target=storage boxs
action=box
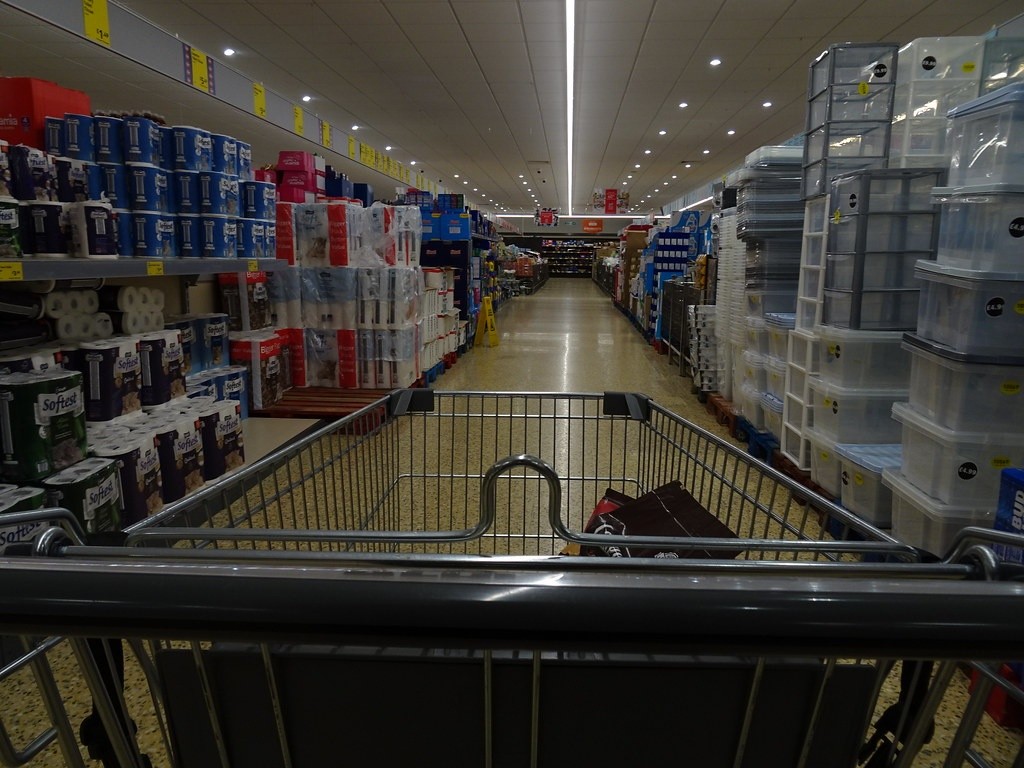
[0,76,92,156]
[254,150,486,321]
[688,36,1024,565]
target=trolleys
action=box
[1,388,1023,768]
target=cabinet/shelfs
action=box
[541,245,595,279]
